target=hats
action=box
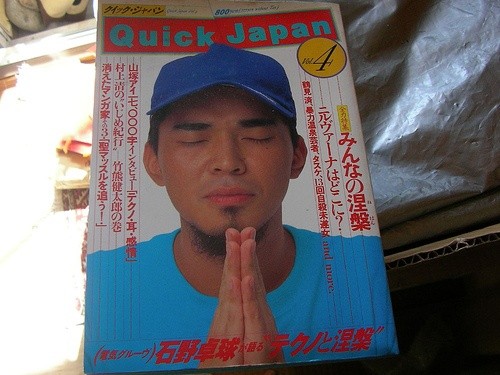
[147,42,298,124]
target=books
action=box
[83,0,400,375]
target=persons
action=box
[83,43,399,375]
